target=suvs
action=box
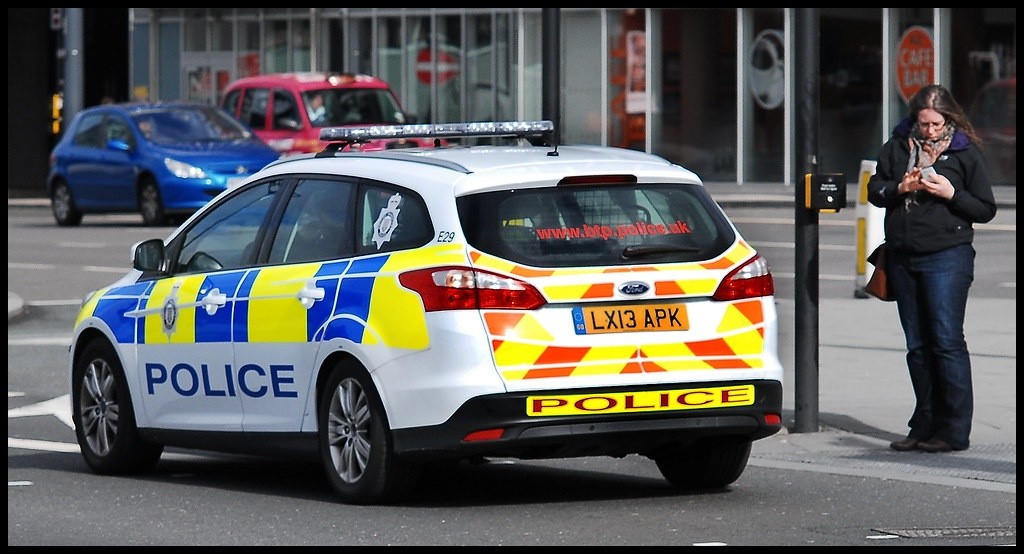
[219,71,452,160]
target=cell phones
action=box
[921,166,938,183]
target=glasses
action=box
[916,118,950,131]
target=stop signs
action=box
[417,49,457,85]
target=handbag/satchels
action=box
[864,242,897,302]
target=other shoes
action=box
[890,437,920,451]
[917,437,957,452]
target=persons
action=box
[868,84,996,453]
[126,117,153,144]
[306,93,326,123]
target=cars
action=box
[46,99,301,229]
[967,77,1015,159]
[67,118,787,506]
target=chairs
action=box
[500,194,546,257]
